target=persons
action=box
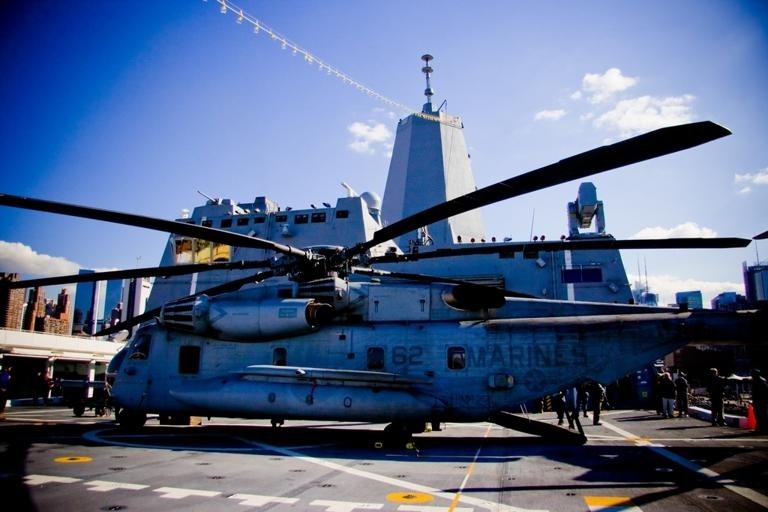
[552,382,609,427]
[653,373,663,416]
[659,372,676,417]
[705,368,727,428]
[674,372,690,418]
[748,370,766,435]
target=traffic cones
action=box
[747,405,758,430]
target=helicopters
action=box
[0,120,752,453]
